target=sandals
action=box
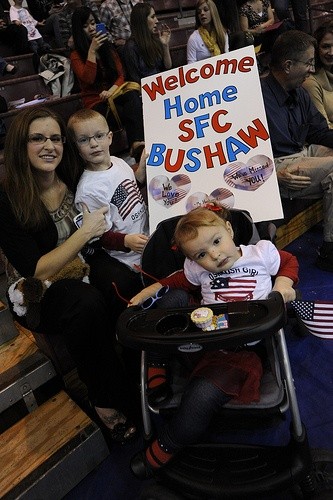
[90,403,138,444]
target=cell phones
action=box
[73,212,99,244]
[96,24,105,37]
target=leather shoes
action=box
[146,362,173,408]
[128,440,187,481]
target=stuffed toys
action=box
[5,255,89,320]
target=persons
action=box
[127,207,300,479]
[0,0,333,163]
[260,30,333,272]
[0,107,144,441]
[66,109,149,278]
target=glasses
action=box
[27,134,66,144]
[290,57,315,69]
[73,130,110,145]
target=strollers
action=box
[117,206,329,497]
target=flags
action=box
[290,299,333,340]
